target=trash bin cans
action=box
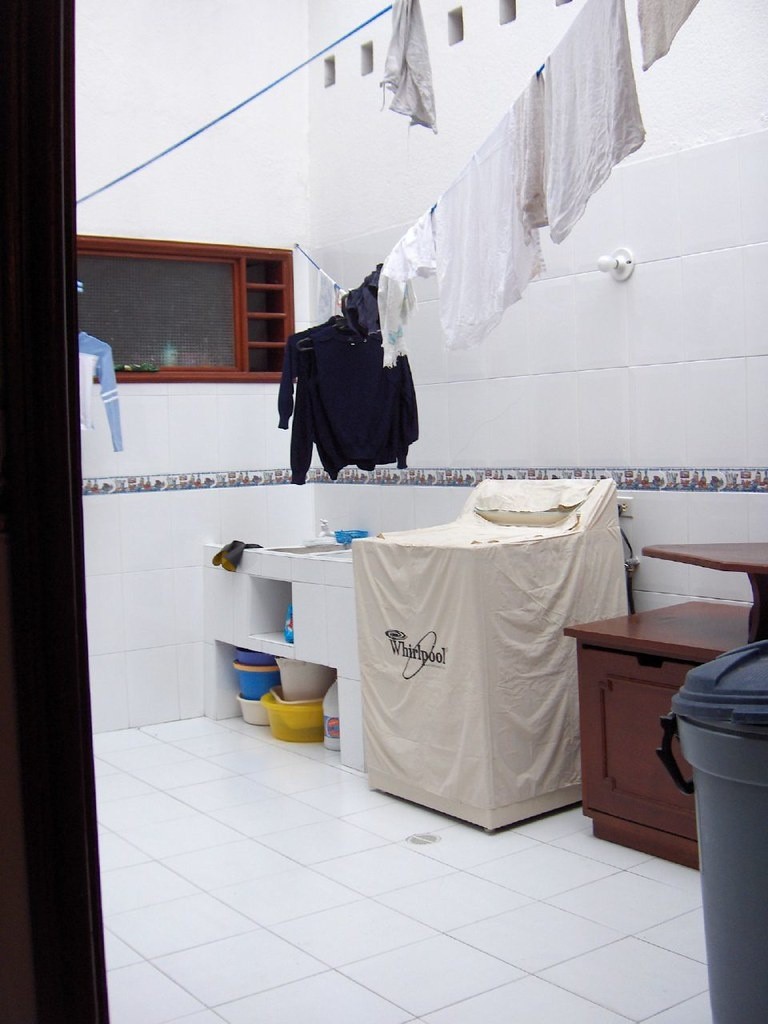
[656,639,768,1024]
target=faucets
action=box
[318,518,336,539]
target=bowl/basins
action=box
[236,687,324,742]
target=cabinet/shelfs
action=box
[563,542,768,869]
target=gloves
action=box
[211,540,264,571]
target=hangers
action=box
[296,288,360,352]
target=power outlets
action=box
[618,496,634,519]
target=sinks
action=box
[264,543,353,554]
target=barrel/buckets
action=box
[656,639,768,1024]
[233,659,282,701]
[322,678,341,750]
[236,647,277,666]
[275,658,337,701]
